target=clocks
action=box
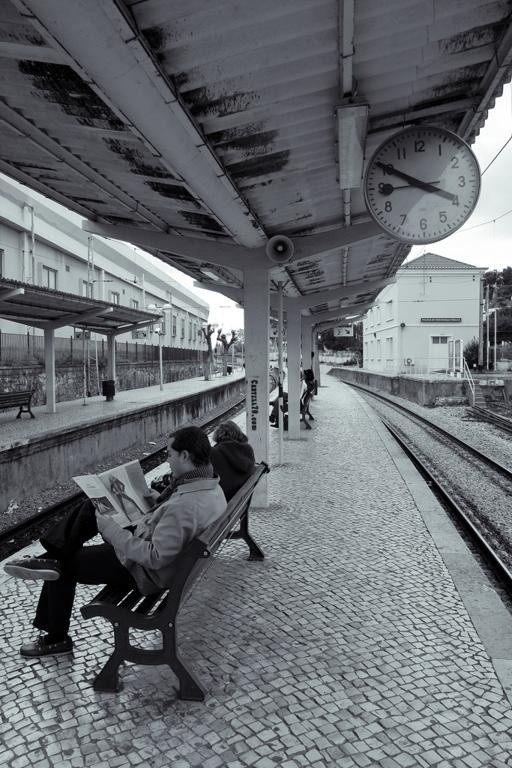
[362,125,481,245]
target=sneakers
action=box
[3,554,61,581]
[20,635,73,658]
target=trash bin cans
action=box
[227,366,233,375]
[102,380,115,401]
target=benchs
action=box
[79,461,268,702]
[0,390,35,419]
[284,385,318,429]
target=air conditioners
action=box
[404,357,416,366]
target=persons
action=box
[3,426,228,657]
[269,358,314,428]
[212,421,255,504]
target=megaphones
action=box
[266,235,295,264]
